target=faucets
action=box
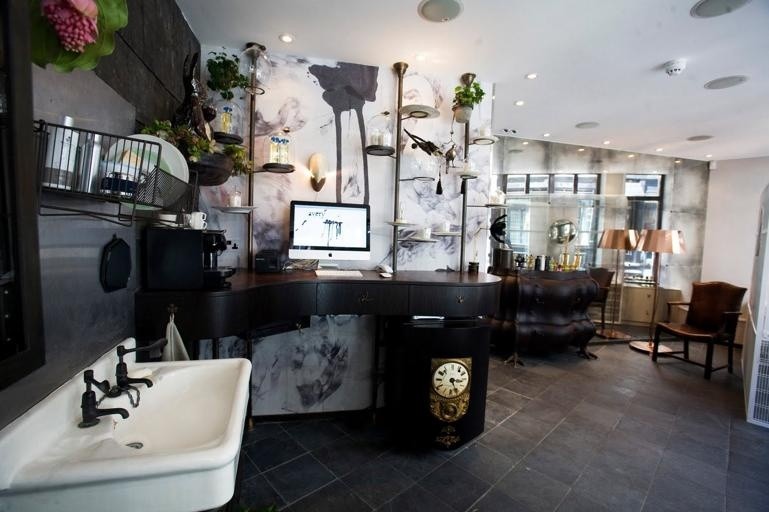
[77,369,129,428]
[113,338,167,388]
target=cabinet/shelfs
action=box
[132,267,503,453]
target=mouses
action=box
[380,273,393,277]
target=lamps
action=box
[417,1,464,25]
[597,227,641,341]
[690,0,751,20]
[704,74,750,90]
[630,228,690,358]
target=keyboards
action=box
[315,270,363,277]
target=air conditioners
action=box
[743,186,768,427]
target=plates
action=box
[186,227,227,234]
[399,104,441,120]
[432,232,462,237]
[212,207,259,215]
[411,237,441,243]
[385,222,418,228]
[103,134,190,212]
[472,136,499,146]
[458,170,479,176]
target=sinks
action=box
[11,358,252,512]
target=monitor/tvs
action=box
[288,200,370,269]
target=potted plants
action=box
[450,81,486,124]
[223,144,249,175]
[204,45,250,101]
[145,118,216,165]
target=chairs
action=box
[588,267,615,331]
[651,278,748,381]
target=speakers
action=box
[255,250,278,272]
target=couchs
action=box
[489,263,599,370]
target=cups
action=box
[464,158,473,171]
[399,202,407,222]
[190,221,208,231]
[479,126,490,135]
[160,214,178,228]
[224,192,241,208]
[191,213,207,221]
[441,221,451,234]
[423,228,433,240]
